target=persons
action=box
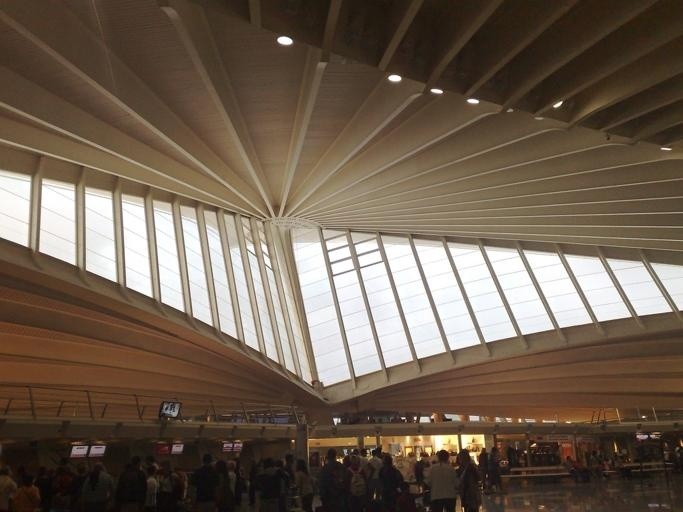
[1,436,508,512]
[509,440,683,490]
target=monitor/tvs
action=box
[649,432,664,441]
[221,440,233,454]
[67,442,90,459]
[171,441,186,455]
[232,440,244,453]
[159,401,182,418]
[155,441,172,455]
[635,433,649,440]
[88,443,107,458]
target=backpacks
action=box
[348,466,366,496]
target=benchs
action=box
[498,464,586,483]
